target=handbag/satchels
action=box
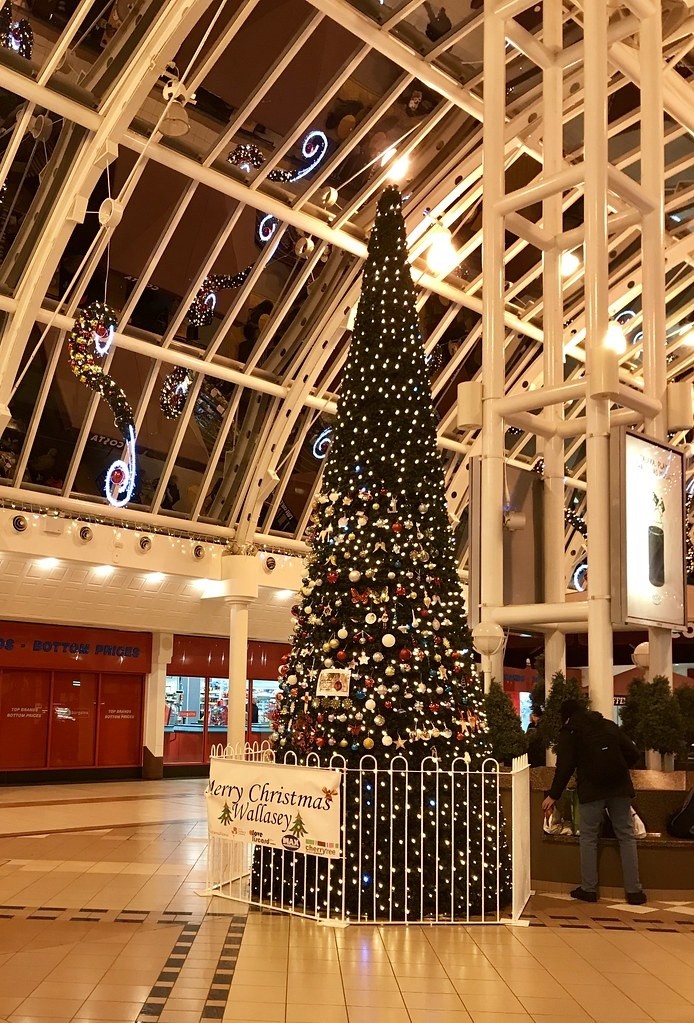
[605,806,647,839]
[542,787,580,836]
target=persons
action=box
[528,712,539,728]
[246,698,258,723]
[540,702,648,905]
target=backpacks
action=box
[666,786,694,839]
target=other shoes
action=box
[627,890,647,905]
[570,887,597,902]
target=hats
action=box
[557,699,578,713]
